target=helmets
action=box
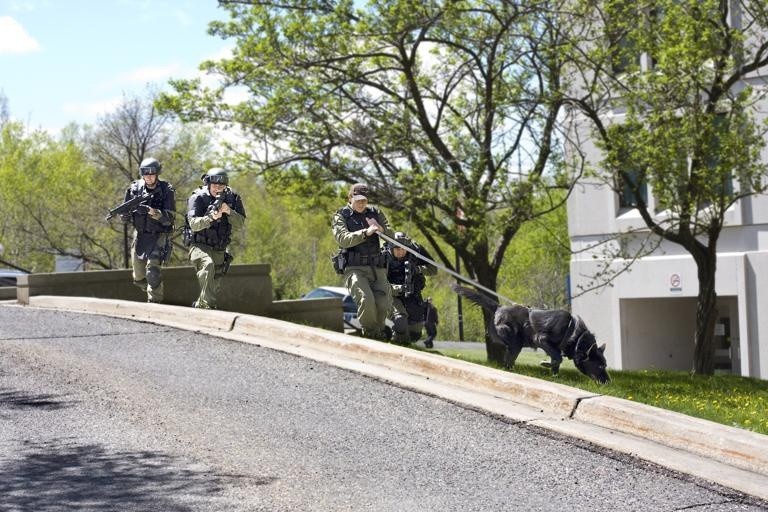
[388,231,411,248]
[139,157,164,175]
[201,167,228,185]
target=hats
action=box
[350,183,369,200]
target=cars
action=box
[304,285,396,341]
[1,269,27,287]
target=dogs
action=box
[449,283,611,385]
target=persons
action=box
[120,158,176,303]
[331,183,393,343]
[187,168,246,309]
[423,296,439,348]
[380,231,436,345]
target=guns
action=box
[106,187,161,221]
[402,261,415,299]
[203,189,226,216]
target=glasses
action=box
[139,167,157,175]
[209,174,229,185]
